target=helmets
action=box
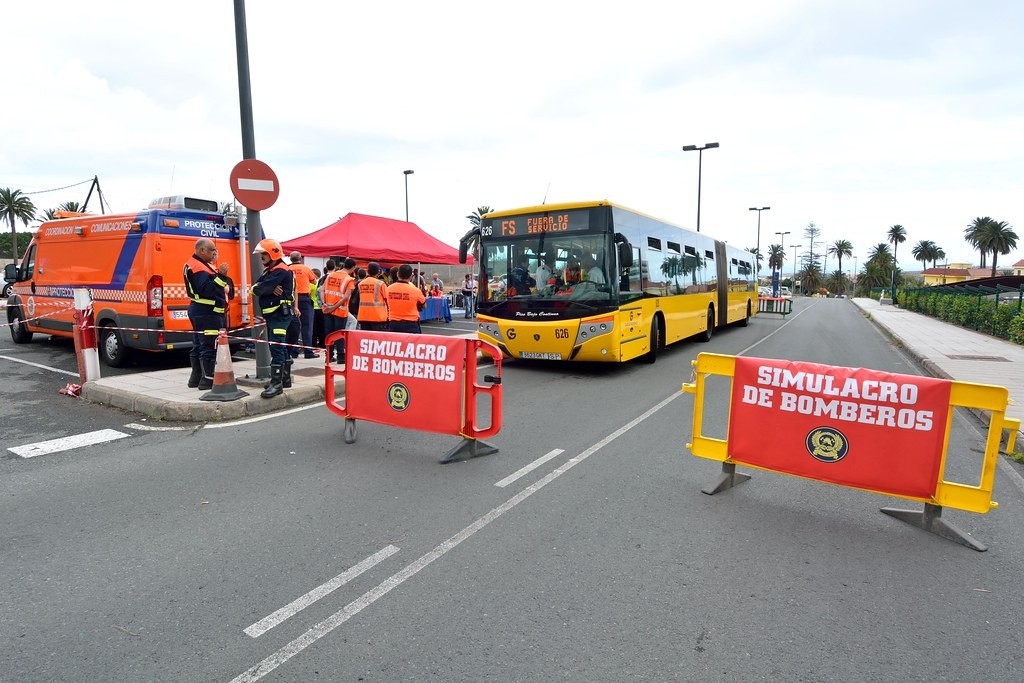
[252,239,283,261]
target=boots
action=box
[264,360,291,389]
[188,359,202,387]
[261,365,284,397]
[198,360,215,390]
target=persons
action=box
[488,281,492,302]
[511,255,536,295]
[462,275,478,319]
[184,239,234,390]
[250,239,301,399]
[286,251,320,359]
[498,275,507,301]
[428,273,444,320]
[310,259,427,364]
[553,256,582,294]
[581,257,606,287]
[576,249,595,278]
[595,247,605,270]
[536,253,562,291]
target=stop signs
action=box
[230,159,280,211]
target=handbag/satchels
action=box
[462,284,472,295]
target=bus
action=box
[458,200,760,366]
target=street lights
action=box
[752,205,771,273]
[791,244,801,290]
[852,255,857,295]
[402,170,417,218]
[683,142,718,231]
[776,229,791,296]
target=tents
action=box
[279,212,474,321]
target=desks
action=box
[419,297,452,324]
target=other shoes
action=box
[292,353,298,358]
[331,357,336,362]
[305,354,319,358]
[465,316,470,319]
[473,316,475,318]
[313,344,318,347]
[320,345,326,348]
[337,361,345,364]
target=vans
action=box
[5,194,272,369]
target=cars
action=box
[757,283,789,296]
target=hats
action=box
[412,269,418,274]
